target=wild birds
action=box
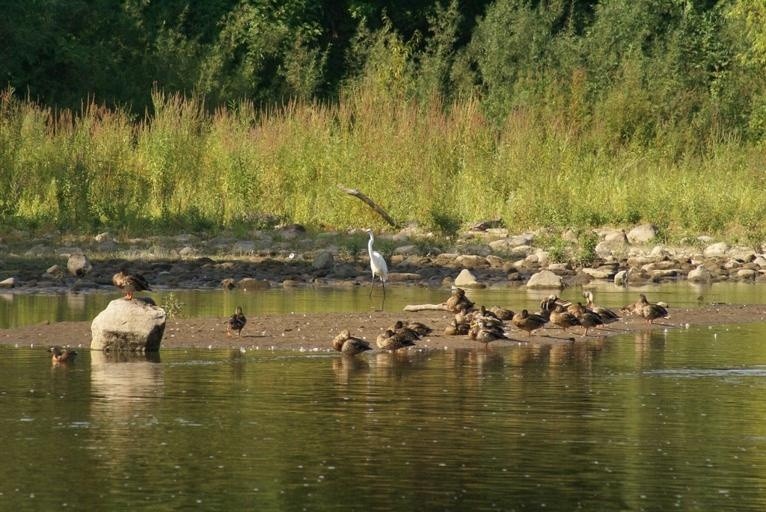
[331,319,432,354]
[45,346,78,361]
[112,272,154,298]
[442,290,668,347]
[362,228,390,313]
[225,304,248,335]
